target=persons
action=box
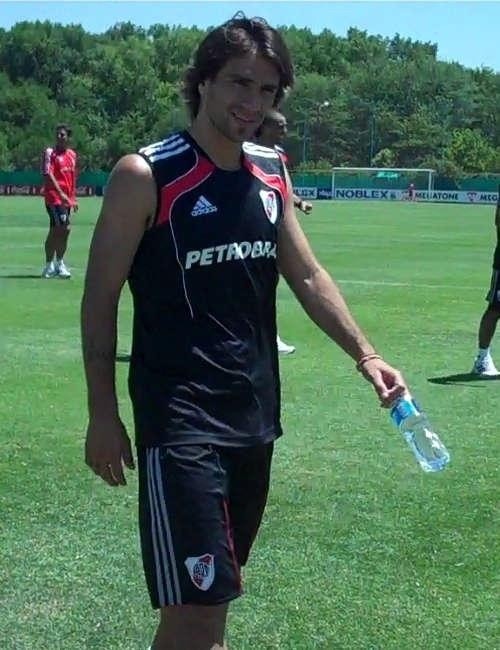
[407,182,415,202]
[471,193,499,378]
[253,108,315,355]
[76,10,416,650]
[41,125,80,279]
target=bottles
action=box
[390,394,451,471]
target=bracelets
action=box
[297,198,302,209]
[355,353,383,372]
[57,187,62,193]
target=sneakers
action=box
[43,268,58,279]
[55,263,71,279]
[277,336,296,353]
[474,355,500,377]
